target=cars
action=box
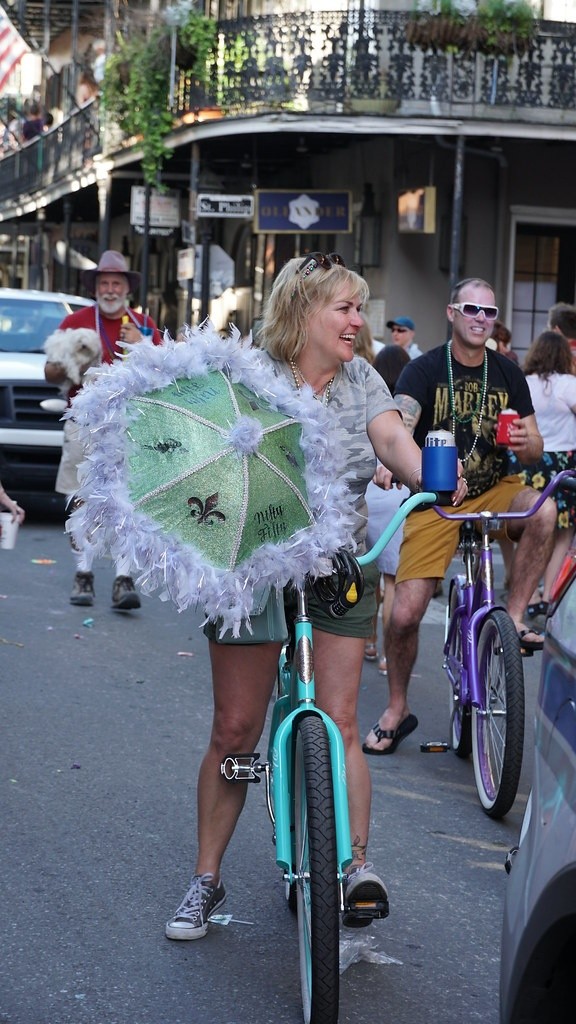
[486,529,576,1024]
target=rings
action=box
[463,479,467,484]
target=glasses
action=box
[450,302,499,320]
[391,328,412,334]
[296,251,347,274]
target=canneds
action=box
[425,429,456,447]
[499,408,518,415]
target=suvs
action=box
[0,290,97,500]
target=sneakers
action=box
[164,873,227,940]
[70,572,96,606]
[341,862,387,928]
[112,575,142,610]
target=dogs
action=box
[44,327,102,394]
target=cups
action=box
[496,408,520,443]
[421,445,459,493]
[0,511,20,550]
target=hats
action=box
[80,249,141,296]
[387,317,415,331]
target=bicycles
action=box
[216,488,445,1024]
[419,466,576,822]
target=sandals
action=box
[377,655,389,676]
[363,643,379,660]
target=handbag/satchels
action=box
[214,578,288,644]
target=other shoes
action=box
[529,599,549,627]
[432,582,443,598]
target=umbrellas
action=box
[64,312,368,638]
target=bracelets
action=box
[408,468,421,490]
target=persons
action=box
[218,329,229,340]
[23,104,43,141]
[0,481,25,524]
[43,112,53,132]
[76,71,102,169]
[365,344,412,675]
[386,316,423,361]
[91,38,106,89]
[0,119,26,160]
[176,326,186,341]
[547,302,576,377]
[489,321,519,364]
[166,257,470,941]
[506,329,576,618]
[45,250,165,612]
[360,274,556,755]
[352,314,375,365]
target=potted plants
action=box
[104,52,138,111]
[162,19,217,73]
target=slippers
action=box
[362,714,419,756]
[493,629,546,655]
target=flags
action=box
[0,7,33,93]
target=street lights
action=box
[193,165,224,326]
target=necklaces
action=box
[95,304,142,360]
[446,339,487,462]
[289,356,334,407]
